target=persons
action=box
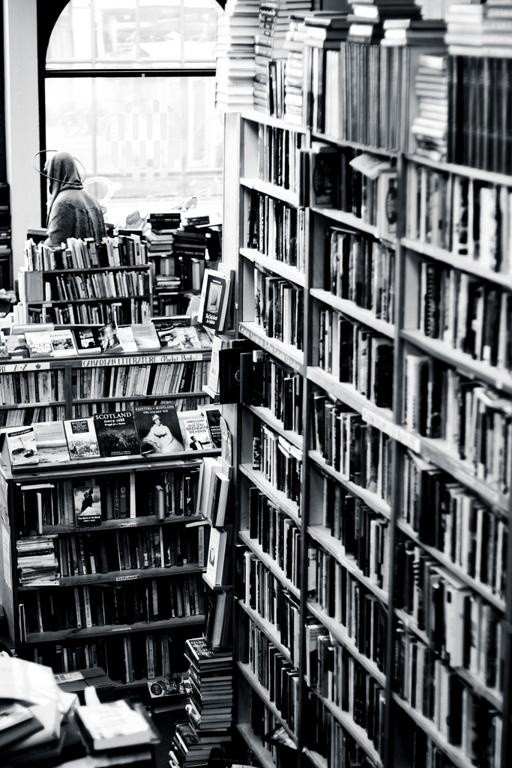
[34,148,106,244]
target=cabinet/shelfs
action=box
[1,105,511,767]
